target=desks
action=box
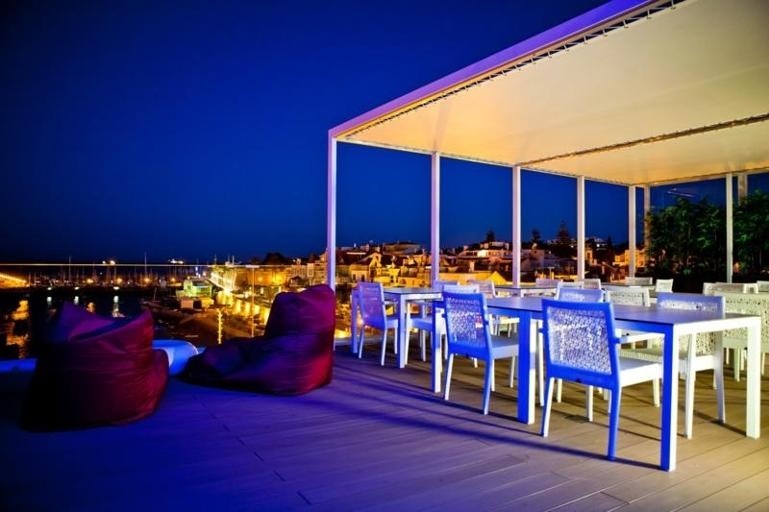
[382,286,443,370]
[430,296,762,473]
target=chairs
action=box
[356,282,400,367]
[439,288,517,417]
[466,276,768,338]
[186,282,339,398]
[411,280,460,361]
[537,282,603,403]
[621,291,726,442]
[540,298,663,462]
[11,305,169,432]
